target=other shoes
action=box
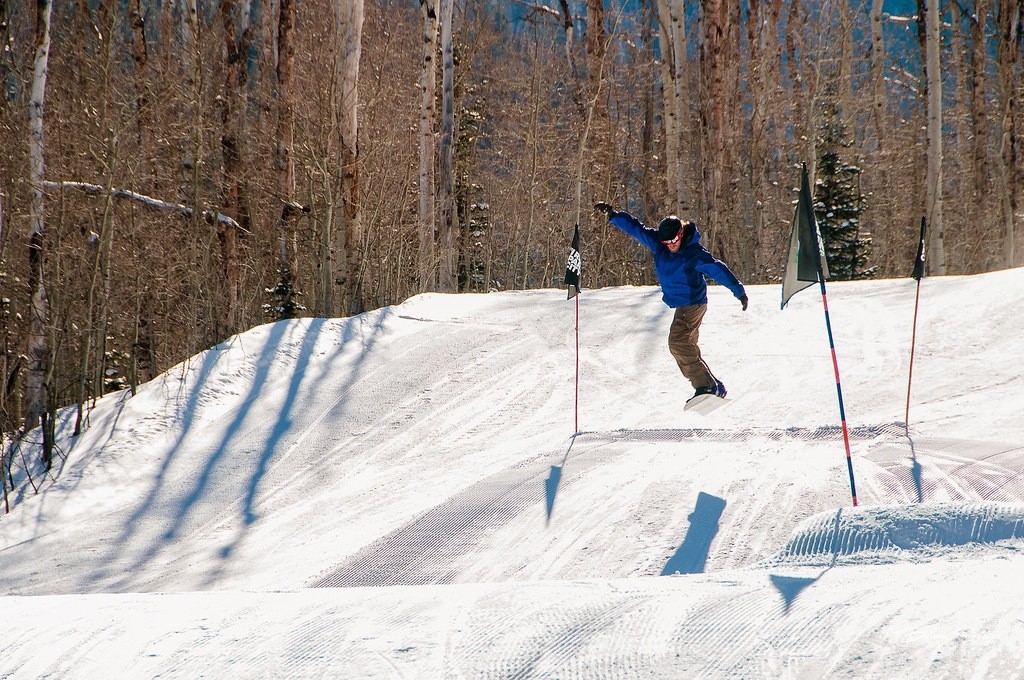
[685,382,727,403]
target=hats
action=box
[657,215,682,240]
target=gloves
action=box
[592,202,613,215]
[738,295,748,311]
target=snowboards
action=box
[682,393,734,417]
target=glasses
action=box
[661,232,681,246]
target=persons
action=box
[593,200,749,403]
